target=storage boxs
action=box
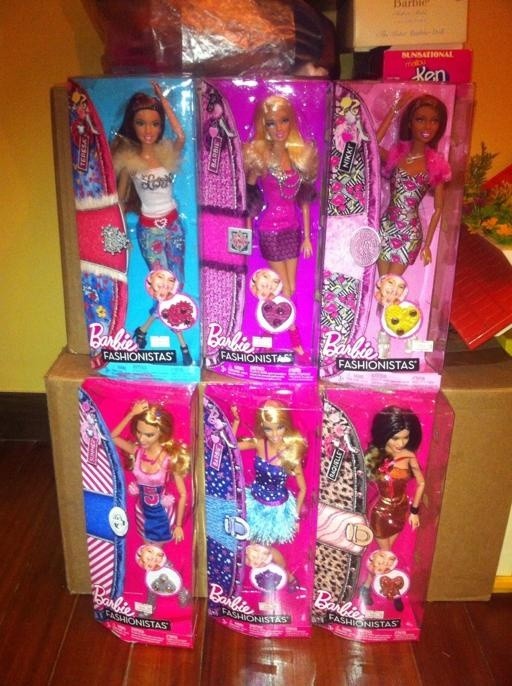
[44,78,478,357]
[43,331,512,605]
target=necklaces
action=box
[141,444,166,465]
[405,151,428,166]
[266,151,305,200]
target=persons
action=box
[369,551,396,575]
[108,398,193,622]
[374,273,406,307]
[239,93,324,369]
[146,270,178,302]
[228,398,313,598]
[136,544,165,571]
[371,84,455,359]
[252,268,281,302]
[360,404,428,611]
[244,544,272,568]
[108,81,198,367]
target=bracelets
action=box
[411,503,419,516]
